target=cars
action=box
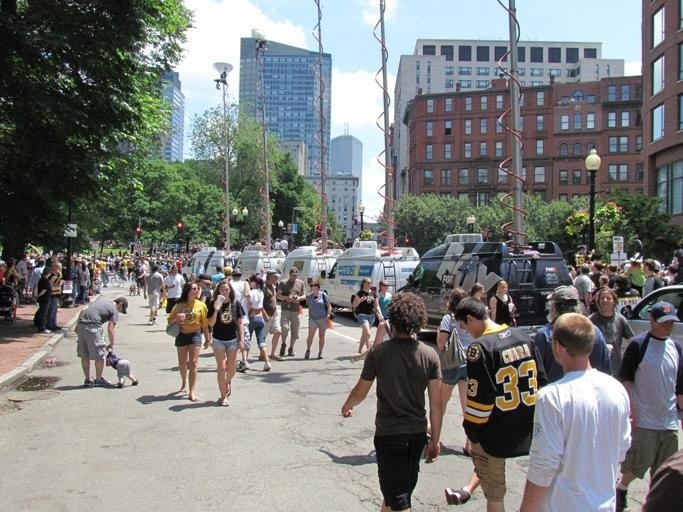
[621,284,683,362]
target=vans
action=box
[399,233,576,331]
[179,239,421,314]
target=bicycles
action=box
[126,274,139,296]
[110,271,125,289]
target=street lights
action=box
[584,149,601,262]
[213,61,233,256]
[290,206,309,250]
[251,30,274,253]
[277,218,285,240]
[232,206,248,250]
[466,213,476,233]
[357,200,365,239]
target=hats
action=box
[651,300,679,324]
[289,266,300,277]
[115,296,129,314]
[266,269,282,278]
[545,283,580,304]
[217,264,241,276]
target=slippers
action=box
[226,379,232,396]
[220,396,229,408]
[180,383,199,403]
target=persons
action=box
[0,233,393,407]
[342,245,682,512]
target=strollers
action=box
[0,285,20,326]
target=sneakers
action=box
[84,376,140,388]
[271,344,326,361]
[263,361,270,371]
[444,485,470,505]
[237,360,250,372]
[148,317,159,327]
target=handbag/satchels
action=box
[166,302,181,338]
[322,291,336,319]
[439,313,468,371]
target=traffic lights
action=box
[177,221,183,230]
[354,216,358,225]
[135,227,141,234]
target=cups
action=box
[214,294,225,310]
[176,313,185,324]
[605,343,614,357]
